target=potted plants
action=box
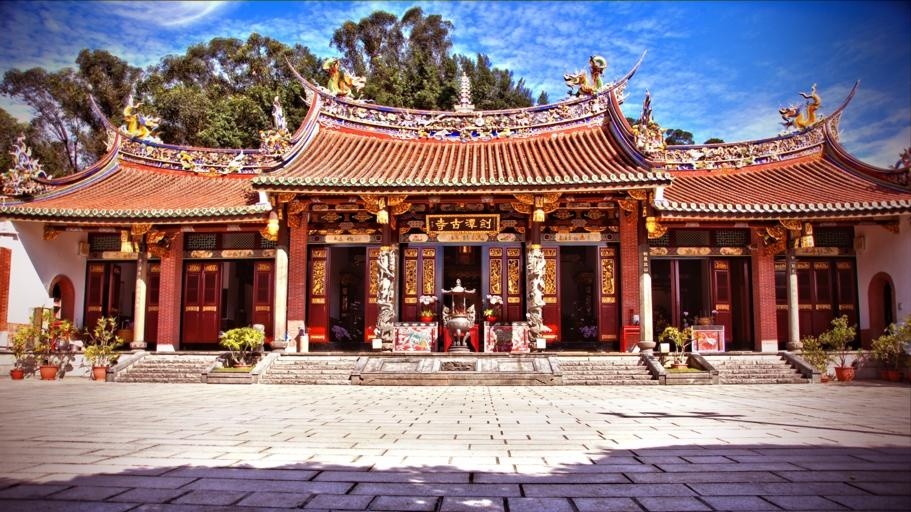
[217,326,267,369]
[801,310,911,383]
[483,308,498,324]
[419,309,433,323]
[7,305,125,382]
[657,323,701,370]
